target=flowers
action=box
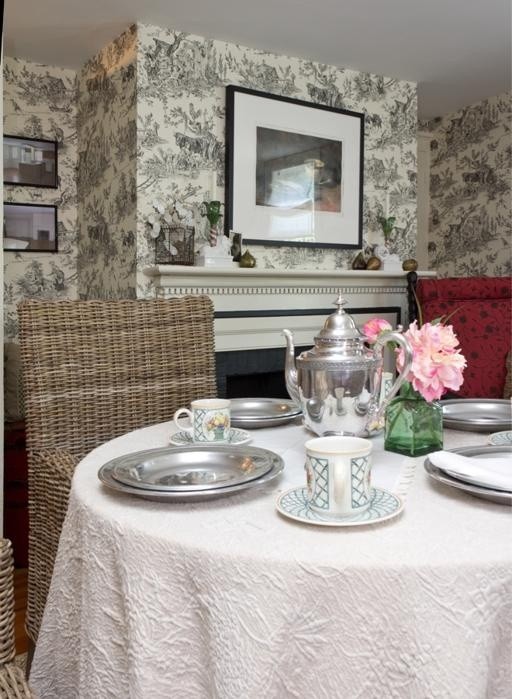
[394,305,468,402]
[360,317,403,345]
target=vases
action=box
[384,382,443,457]
[366,346,396,430]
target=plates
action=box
[423,445,512,506]
[230,412,303,428]
[441,445,512,494]
[274,486,406,528]
[97,443,285,503]
[231,398,303,419]
[488,430,512,445]
[168,427,252,446]
[438,397,512,431]
[112,447,274,491]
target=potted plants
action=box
[376,216,403,270]
[196,201,234,267]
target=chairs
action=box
[17,297,218,645]
[407,271,512,398]
[1,536,28,698]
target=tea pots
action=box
[281,288,413,438]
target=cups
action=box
[172,399,232,445]
[373,372,394,408]
[304,434,370,517]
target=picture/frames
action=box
[4,135,58,188]
[225,84,365,249]
[3,202,57,253]
